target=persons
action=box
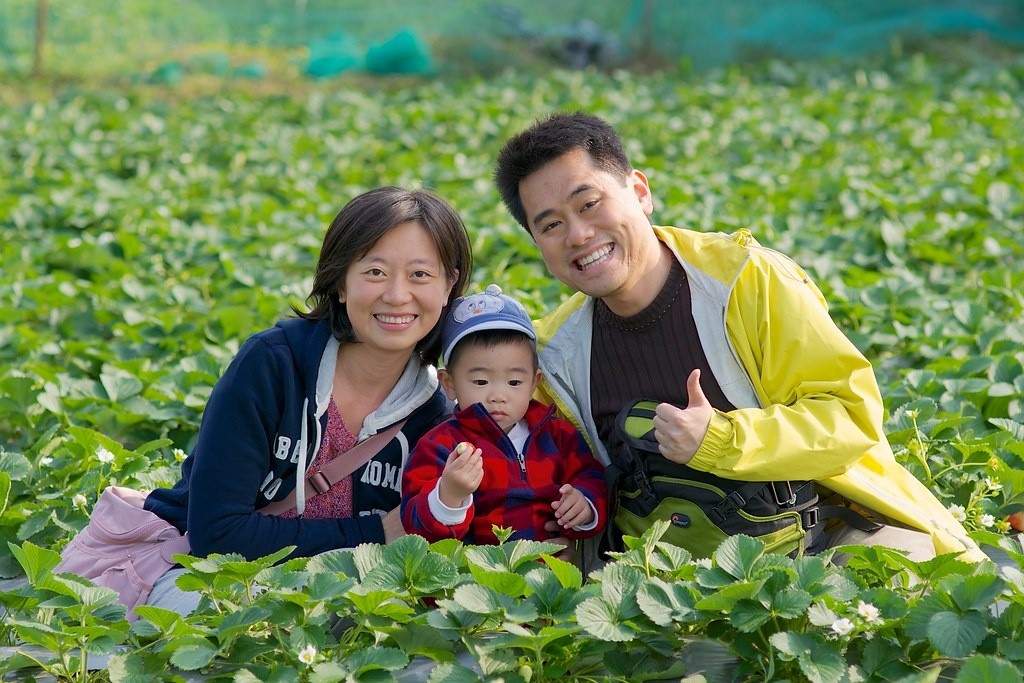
[492,110,1023,616]
[138,185,474,616]
[400,282,608,586]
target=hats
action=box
[441,284,537,371]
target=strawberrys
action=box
[457,442,477,456]
[1009,511,1024,532]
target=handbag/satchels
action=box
[591,397,883,561]
[48,485,186,623]
